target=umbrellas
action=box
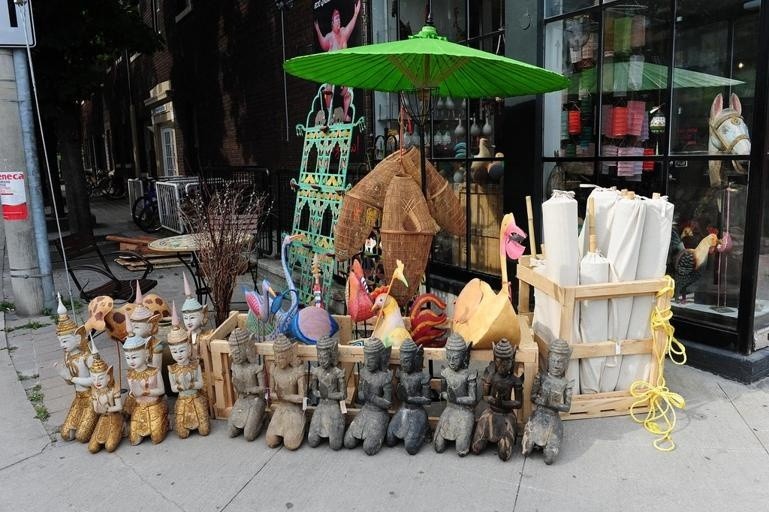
[282,25,571,324]
[562,61,744,104]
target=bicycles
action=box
[82,166,194,235]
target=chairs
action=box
[55,233,158,344]
[204,214,259,312]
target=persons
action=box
[266,334,347,451]
[57,293,215,453]
[229,329,266,441]
[433,332,524,461]
[344,337,433,456]
[521,339,571,465]
[313,1,364,125]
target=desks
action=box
[147,229,255,307]
